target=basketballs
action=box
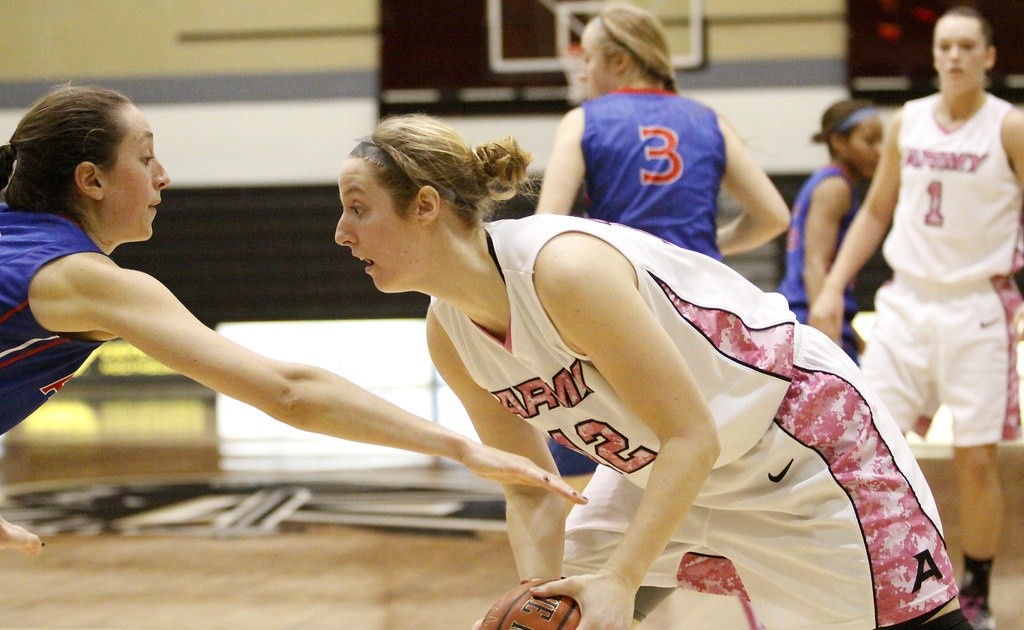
[478,578,582,630]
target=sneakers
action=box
[959,594,995,629]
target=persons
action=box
[334,115,974,630]
[534,6,1024,630]
[0,84,588,555]
